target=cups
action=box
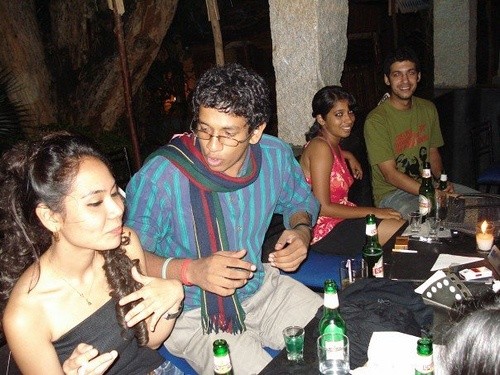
[408,211,422,232]
[339,259,369,288]
[317,333,350,375]
[474,223,497,253]
[282,325,305,361]
[426,217,440,237]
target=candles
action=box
[475,221,495,253]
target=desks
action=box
[258,211,500,375]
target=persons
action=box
[0,131,186,375]
[124,63,325,375]
[364,54,483,225]
[442,289,500,375]
[300,86,408,264]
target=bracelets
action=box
[77,367,84,375]
[161,257,176,279]
[181,259,192,286]
[162,300,184,319]
[293,223,311,232]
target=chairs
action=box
[148,120,500,375]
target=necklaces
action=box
[52,267,94,305]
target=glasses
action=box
[188,114,253,148]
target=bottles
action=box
[438,168,448,190]
[212,339,235,375]
[318,278,347,368]
[415,337,435,375]
[418,162,436,223]
[362,214,385,280]
